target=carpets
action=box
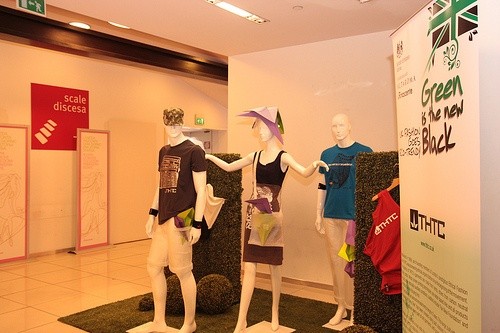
[57,286,351,333]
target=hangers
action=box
[372,164,399,200]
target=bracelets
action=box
[192,220,203,229]
[149,208,159,217]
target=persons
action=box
[146,108,208,333]
[315,114,375,326]
[205,106,330,333]
